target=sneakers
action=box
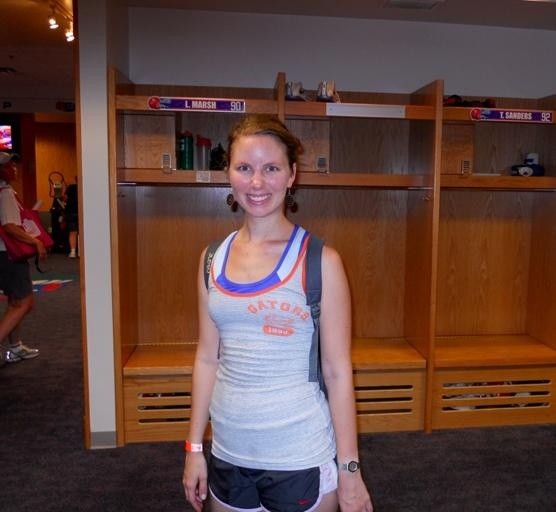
[67,248,76,258]
[286,80,313,102]
[0,340,40,364]
[315,80,341,103]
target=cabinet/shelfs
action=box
[105,62,555,452]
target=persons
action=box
[0,149,49,366]
[63,175,79,259]
[180,115,375,512]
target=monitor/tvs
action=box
[0,125,14,152]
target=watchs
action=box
[337,460,362,473]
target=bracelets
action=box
[184,439,204,452]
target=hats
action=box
[0,151,20,165]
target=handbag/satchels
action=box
[0,186,55,260]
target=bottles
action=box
[178,131,226,170]
